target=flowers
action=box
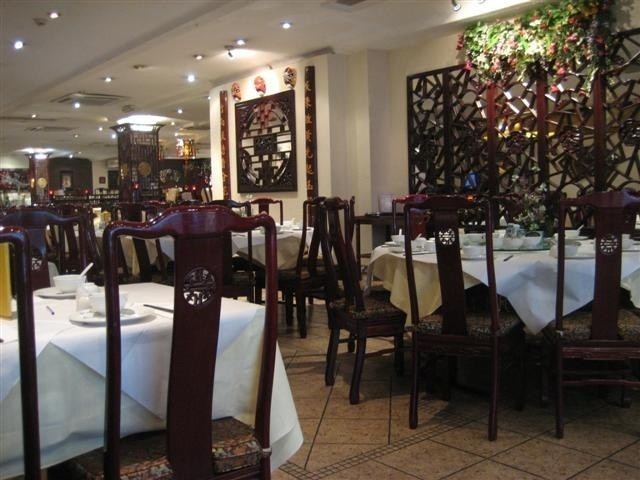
[456,0,624,101]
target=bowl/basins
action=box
[385,234,436,252]
[492,236,541,250]
[258,220,312,234]
[53,274,86,293]
[552,245,577,256]
[565,230,580,237]
[462,232,486,255]
[90,291,128,316]
[621,233,633,249]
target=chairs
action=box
[540,190,640,438]
[47,205,278,480]
[318,197,404,405]
[1,197,283,297]
[0,227,42,480]
[392,193,527,441]
[279,196,356,340]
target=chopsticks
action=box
[144,304,174,314]
[403,251,429,256]
[504,254,514,261]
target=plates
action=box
[460,254,497,260]
[35,283,98,299]
[566,235,589,240]
[555,252,596,259]
[622,241,640,251]
[69,307,155,325]
